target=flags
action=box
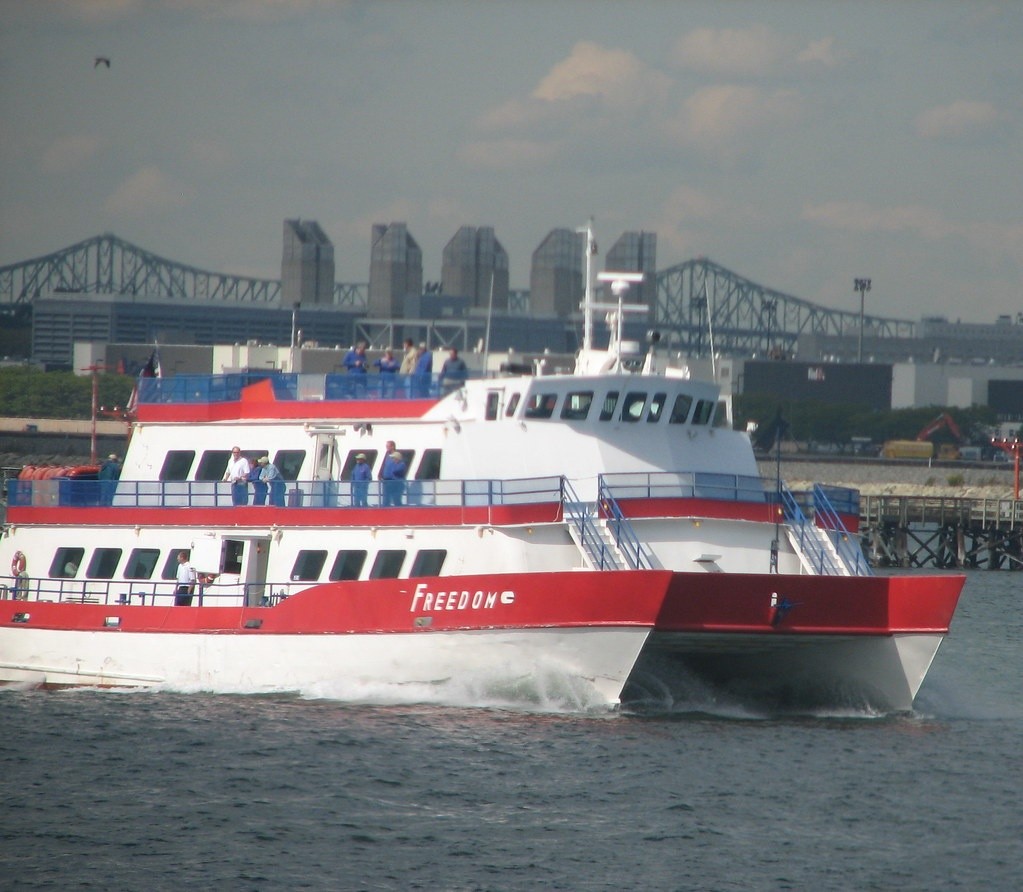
[128,348,161,416]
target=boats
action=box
[0,271,965,723]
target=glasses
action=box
[232,452,240,454]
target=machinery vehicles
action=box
[877,410,967,463]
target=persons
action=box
[97,453,119,507]
[350,453,371,506]
[399,338,433,399]
[223,446,250,506]
[242,458,268,506]
[343,339,368,394]
[374,345,399,400]
[379,440,405,505]
[258,457,286,507]
[438,349,468,392]
[172,551,196,606]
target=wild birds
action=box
[94,57,112,68]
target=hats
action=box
[258,456,270,463]
[355,453,367,460]
[384,346,393,352]
[389,452,401,457]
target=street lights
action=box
[852,277,871,438]
[763,298,780,360]
[574,226,594,349]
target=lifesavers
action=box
[16,571,29,598]
[12,551,25,576]
[197,573,215,584]
